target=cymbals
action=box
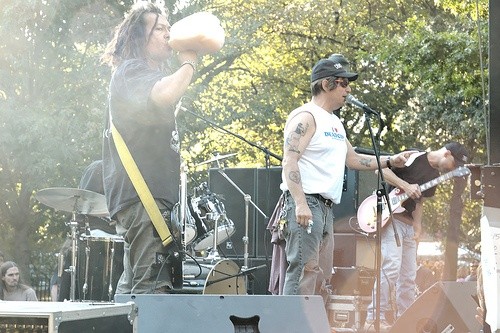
[194,153,238,167]
[35,187,110,215]
[79,228,125,243]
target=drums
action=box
[181,257,247,296]
[170,202,197,246]
[187,193,235,251]
[69,237,122,300]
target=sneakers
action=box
[364,320,392,332]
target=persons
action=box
[0,261,38,301]
[99,2,199,294]
[268,53,419,309]
[415,257,479,294]
[49,160,128,302]
[364,142,468,333]
[476,263,484,326]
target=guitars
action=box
[357,167,471,233]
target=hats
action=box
[311,59,358,82]
[445,141,472,165]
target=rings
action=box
[306,220,314,234]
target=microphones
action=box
[345,95,378,116]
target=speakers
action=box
[389,280,484,332]
[207,166,387,329]
[113,291,334,333]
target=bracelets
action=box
[181,61,195,73]
[387,156,392,169]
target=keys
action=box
[278,202,288,232]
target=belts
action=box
[307,193,335,209]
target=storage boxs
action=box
[324,295,373,333]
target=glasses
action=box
[334,80,350,88]
